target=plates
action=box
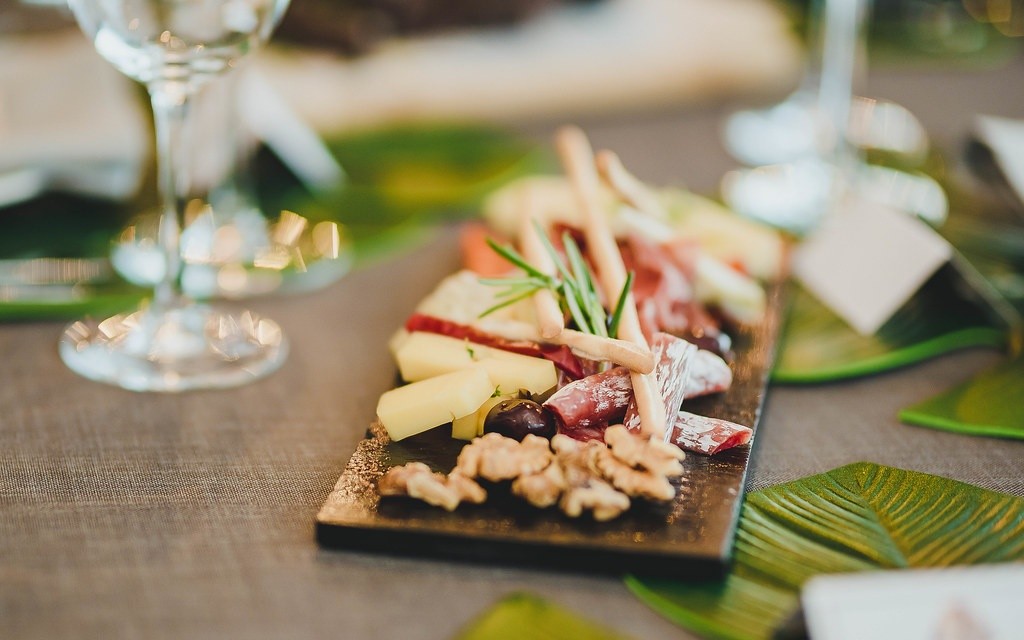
[313,271,779,582]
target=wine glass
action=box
[53,0,346,395]
[721,4,947,236]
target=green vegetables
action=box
[479,216,636,373]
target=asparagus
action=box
[495,123,684,440]
